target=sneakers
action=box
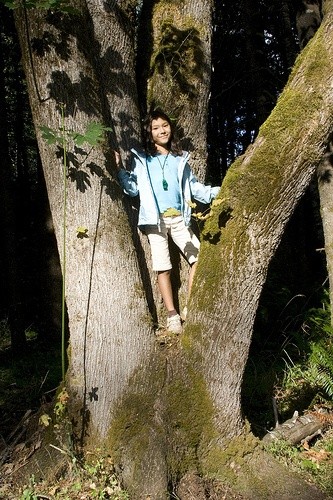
[166,314,182,335]
[182,306,189,321]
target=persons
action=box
[113,108,220,334]
